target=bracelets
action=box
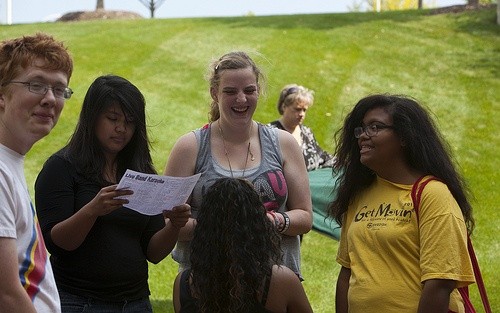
[269,209,291,234]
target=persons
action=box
[33,76,193,313]
[161,52,314,313]
[326,94,477,313]
[269,83,340,172]
[0,33,74,313]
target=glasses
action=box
[9,80,73,100]
[283,88,306,102]
[355,123,396,138]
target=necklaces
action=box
[217,125,255,178]
